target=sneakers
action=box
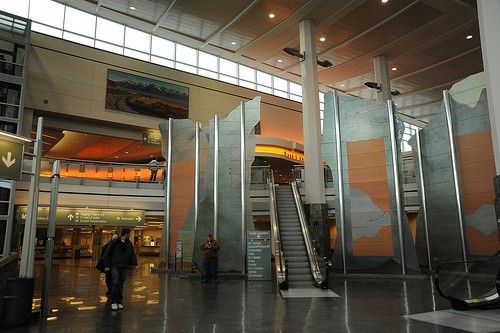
[111,303,123,310]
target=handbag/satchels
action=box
[95,259,111,273]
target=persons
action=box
[95,228,139,310]
[199,233,222,284]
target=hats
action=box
[208,233,213,237]
